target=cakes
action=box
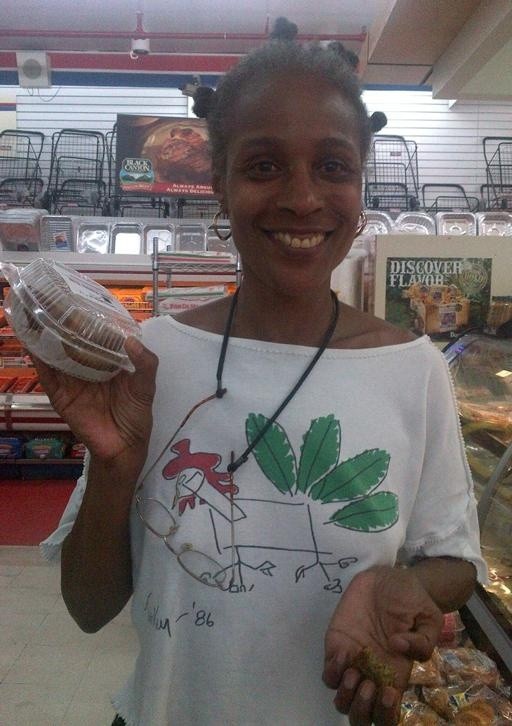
[404,283,469,337]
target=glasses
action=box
[130,387,236,593]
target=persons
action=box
[28,17,491,725]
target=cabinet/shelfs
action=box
[1,251,242,464]
[399,327,512,675]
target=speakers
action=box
[16,49,52,87]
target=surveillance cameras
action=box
[130,36,150,55]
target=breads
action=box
[395,554,512,726]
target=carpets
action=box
[0,478,77,546]
